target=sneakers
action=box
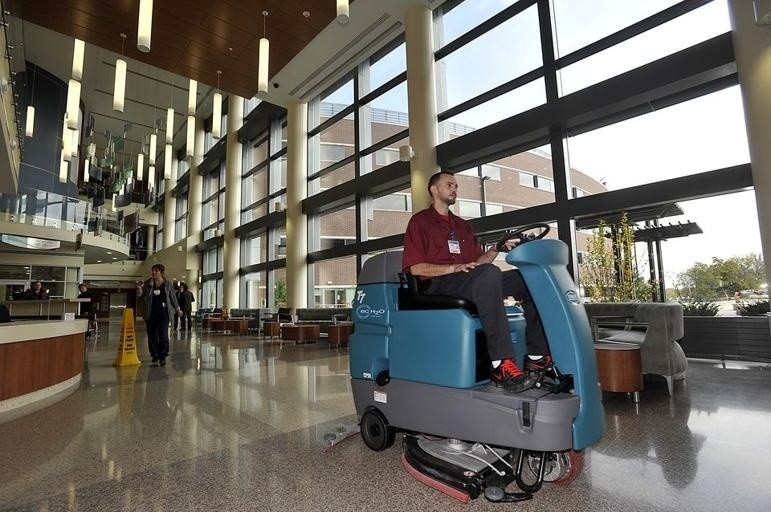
[152,356,167,366]
[523,354,552,388]
[487,357,535,393]
[173,326,191,332]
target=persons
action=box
[136,264,184,366]
[136,365,181,449]
[173,285,185,330]
[170,330,184,371]
[24,280,51,300]
[181,330,193,375]
[77,283,98,340]
[401,170,554,393]
[179,285,196,331]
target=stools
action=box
[593,343,646,404]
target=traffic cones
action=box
[113,308,141,365]
[113,365,141,419]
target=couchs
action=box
[185,300,354,352]
[582,299,689,398]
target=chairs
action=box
[396,271,479,317]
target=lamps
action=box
[212,73,222,139]
[258,12,270,96]
[138,1,153,52]
[127,170,133,186]
[119,184,125,196]
[147,166,156,191]
[137,132,144,180]
[113,36,126,112]
[83,160,89,185]
[336,0,349,25]
[164,81,178,180]
[148,97,156,164]
[187,79,199,157]
[59,39,86,184]
[19,64,39,142]
[112,194,116,211]
